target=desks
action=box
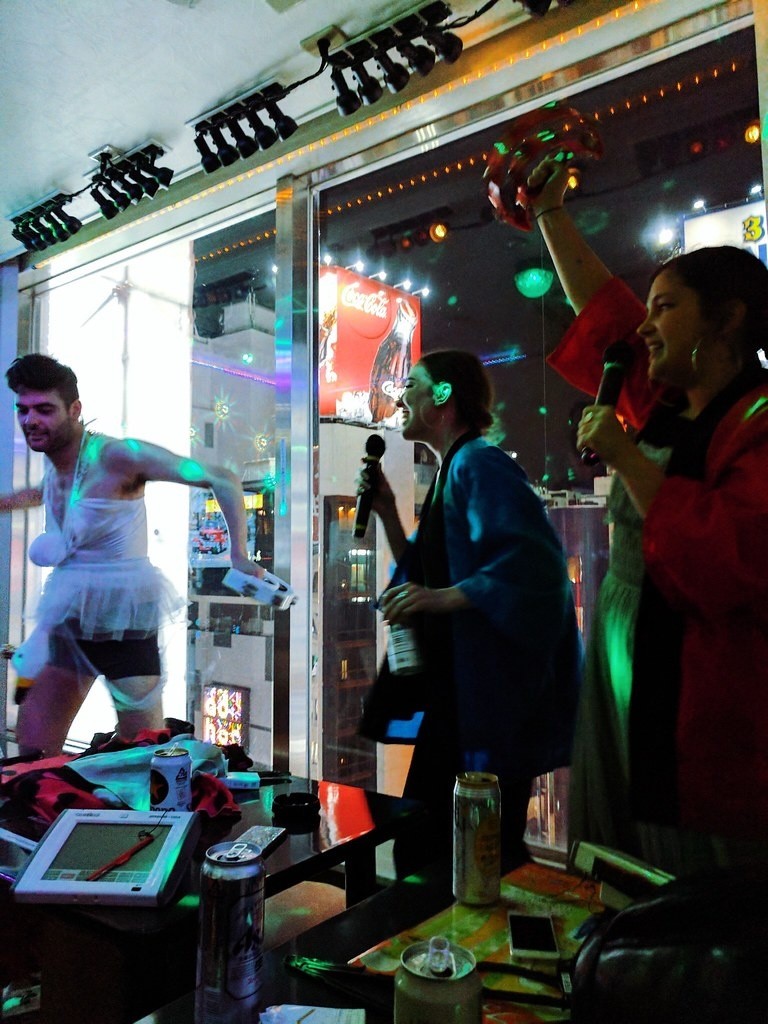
[259,870,596,1024]
[16,759,426,981]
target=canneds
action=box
[195,841,263,999]
[149,742,192,812]
[451,771,501,905]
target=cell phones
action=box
[507,910,561,966]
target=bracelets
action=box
[536,206,563,220]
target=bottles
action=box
[386,582,419,676]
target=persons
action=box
[524,152,768,891]
[1,352,261,756]
[357,349,580,878]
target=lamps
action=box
[5,2,463,252]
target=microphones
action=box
[352,434,386,539]
[580,338,637,467]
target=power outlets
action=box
[298,27,346,59]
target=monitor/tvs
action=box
[10,805,204,904]
